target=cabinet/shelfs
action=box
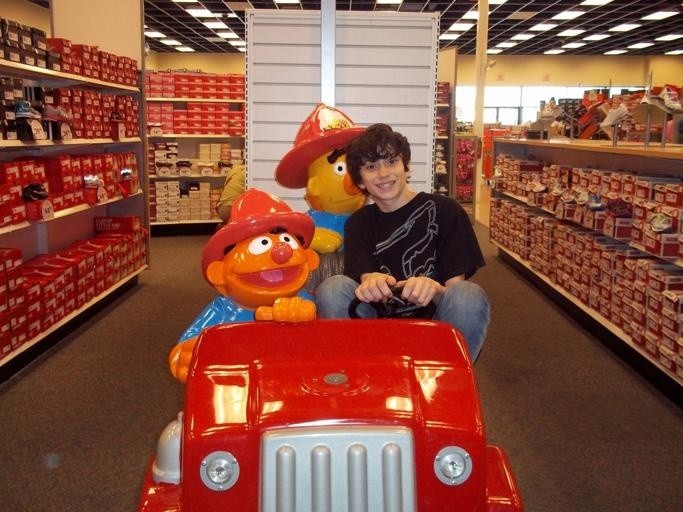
[145,96,246,228]
[487,94,681,387]
[0,57,149,368]
[438,102,522,204]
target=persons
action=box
[315,123,491,368]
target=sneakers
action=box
[111,112,125,122]
[15,101,69,122]
[640,96,649,104]
[658,87,683,112]
[600,103,631,128]
[84,174,103,188]
[494,168,502,176]
[121,169,134,180]
[24,182,49,200]
[648,213,672,233]
[525,179,604,211]
